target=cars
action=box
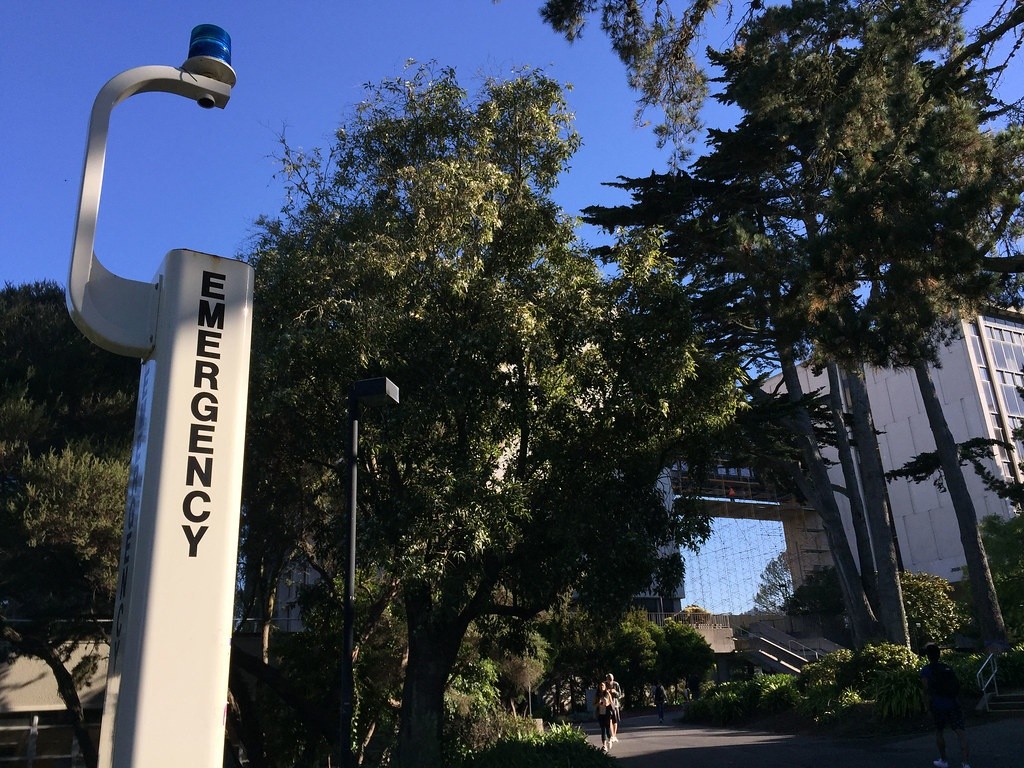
[674,604,710,624]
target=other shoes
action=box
[603,745,607,751]
[614,737,618,742]
[608,742,612,749]
[611,737,615,743]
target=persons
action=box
[601,673,624,742]
[652,679,667,723]
[591,680,618,753]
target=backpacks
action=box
[655,685,662,697]
